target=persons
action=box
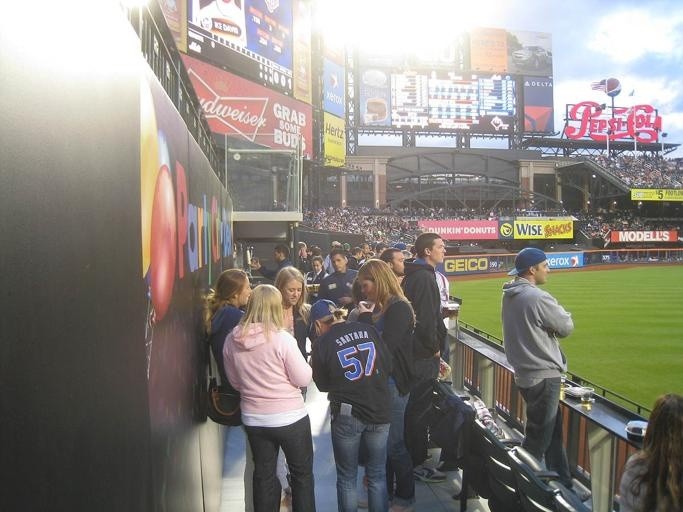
[352,259,417,511]
[206,267,293,511]
[273,265,318,404]
[307,298,393,512]
[222,284,315,511]
[397,231,448,482]
[499,246,575,490]
[617,392,683,512]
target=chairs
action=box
[429,379,593,511]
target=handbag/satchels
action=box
[205,376,244,427]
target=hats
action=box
[507,248,546,277]
[309,299,338,333]
[394,243,407,252]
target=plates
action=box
[624,427,643,442]
[565,390,590,401]
[565,382,571,389]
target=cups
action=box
[559,374,567,398]
[448,302,459,321]
[360,301,373,309]
[579,386,594,410]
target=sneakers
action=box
[414,463,447,484]
[358,497,415,511]
